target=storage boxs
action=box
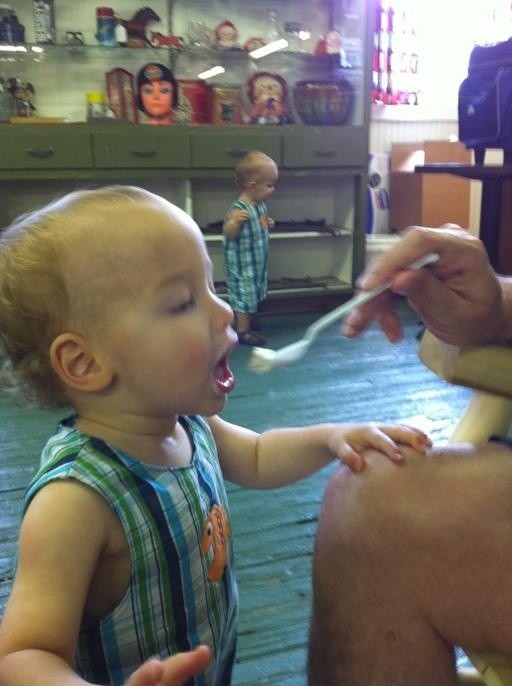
[389,139,472,230]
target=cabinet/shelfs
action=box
[0,0,376,317]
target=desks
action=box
[415,164,512,276]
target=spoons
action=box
[250,253,441,375]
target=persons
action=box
[305,219,511,686]
[220,149,278,348]
[1,178,433,686]
[136,62,178,127]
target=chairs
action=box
[417,328,512,686]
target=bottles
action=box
[87,92,106,121]
[267,10,302,52]
[98,7,115,47]
